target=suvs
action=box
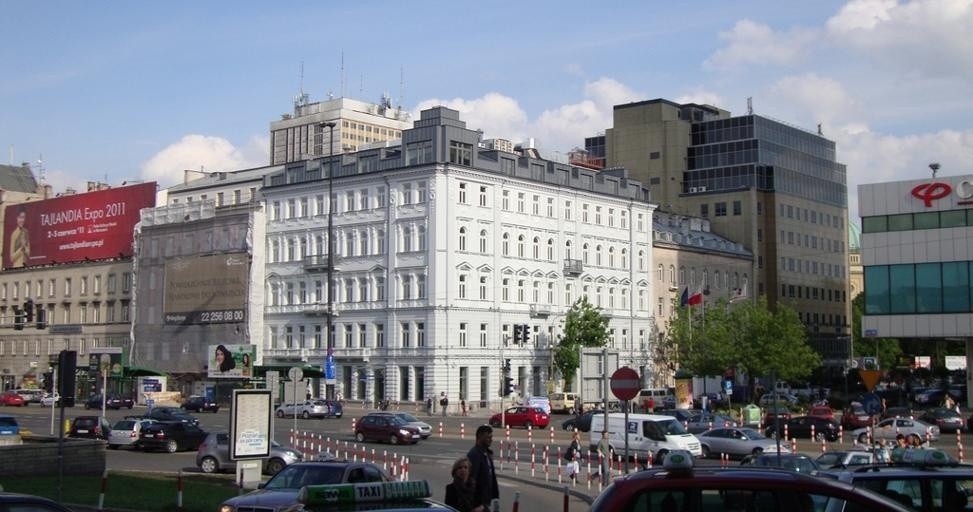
[1,415,23,445]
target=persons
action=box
[444,456,490,512]
[460,397,468,416]
[590,431,611,486]
[10,204,31,267]
[439,392,449,416]
[241,353,250,376]
[214,345,236,372]
[647,397,654,413]
[565,432,584,484]
[573,395,580,416]
[466,425,500,506]
[304,378,346,408]
[515,393,524,405]
[867,393,956,462]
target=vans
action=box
[197,430,303,476]
[71,407,208,453]
[734,381,830,405]
[590,412,702,465]
[527,393,581,418]
[182,396,220,413]
[357,411,432,445]
[1,387,60,408]
[639,388,675,408]
[278,399,344,418]
[85,394,134,411]
[697,393,720,401]
[489,406,550,429]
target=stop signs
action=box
[611,368,640,400]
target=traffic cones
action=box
[64,418,70,439]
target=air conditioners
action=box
[689,186,696,192]
[698,186,706,191]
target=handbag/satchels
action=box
[564,447,572,461]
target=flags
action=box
[689,284,702,306]
[680,286,688,306]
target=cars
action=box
[563,409,611,432]
[218,452,459,511]
[764,401,876,441]
[851,414,939,447]
[882,407,972,432]
[877,382,965,408]
[681,409,788,459]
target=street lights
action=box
[836,336,853,366]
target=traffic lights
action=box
[505,377,513,396]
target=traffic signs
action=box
[864,329,877,337]
[325,363,336,385]
